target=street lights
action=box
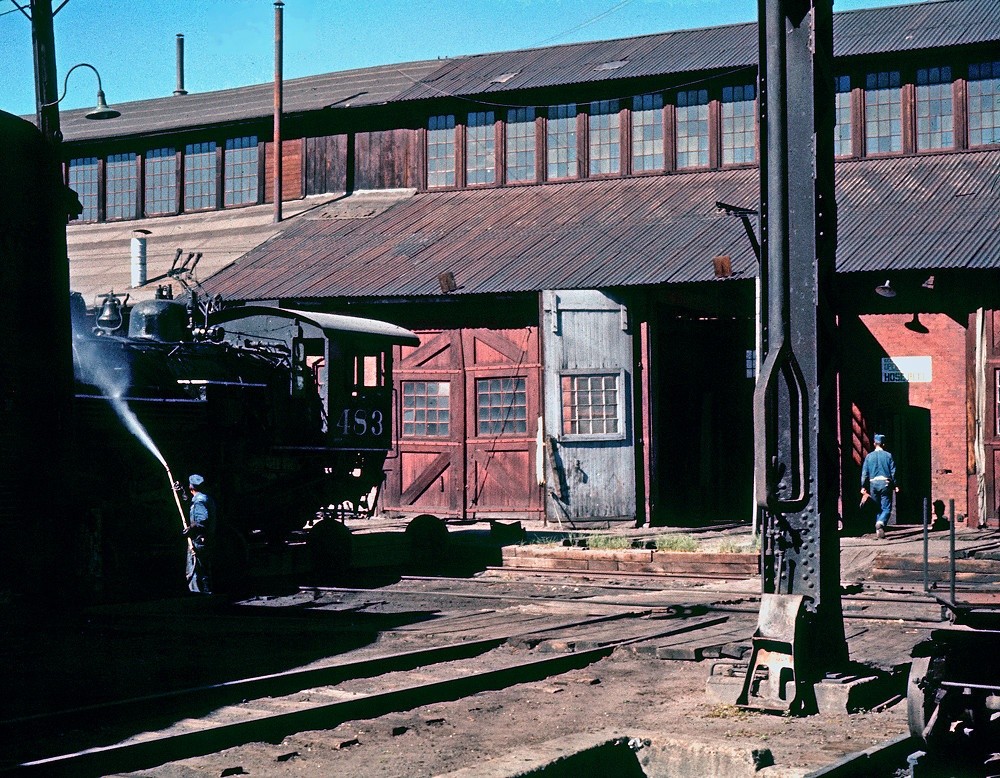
[31,0,122,292]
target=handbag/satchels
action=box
[859,493,871,508]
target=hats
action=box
[874,435,885,443]
[189,474,205,490]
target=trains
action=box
[66,288,418,545]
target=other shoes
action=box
[875,523,884,539]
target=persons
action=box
[173,474,215,597]
[860,434,900,538]
